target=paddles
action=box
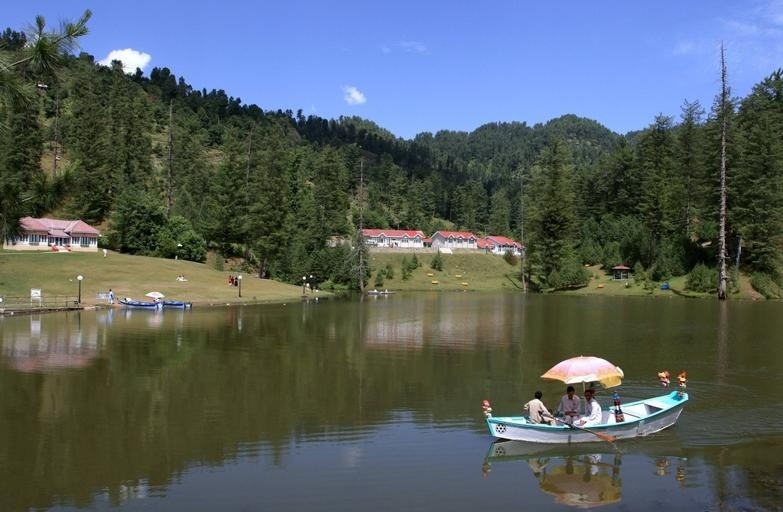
[539,412,617,443]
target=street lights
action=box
[177,243,183,260]
[238,275,242,297]
[302,275,314,293]
[76,275,84,303]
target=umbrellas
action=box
[538,463,622,509]
[144,291,166,298]
[540,354,624,415]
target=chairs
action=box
[31,288,44,308]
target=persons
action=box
[103,248,108,257]
[523,390,557,426]
[554,385,582,424]
[108,289,114,304]
[527,457,551,478]
[174,254,178,260]
[573,388,603,426]
[235,277,239,286]
[229,275,232,286]
[557,455,579,475]
[231,277,234,284]
[176,273,185,281]
[124,297,132,303]
[582,454,603,483]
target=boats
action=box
[481,355,689,444]
[368,289,395,295]
[119,298,192,309]
[482,434,688,483]
